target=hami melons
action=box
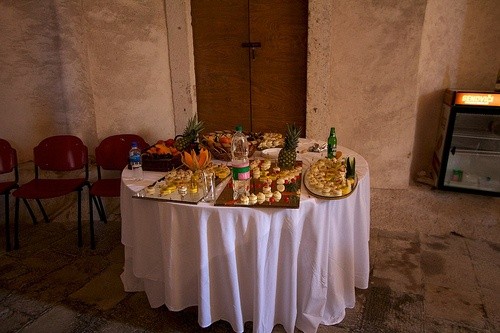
[181,148,212,170]
[146,142,178,154]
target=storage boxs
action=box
[141,141,199,172]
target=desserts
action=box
[307,158,350,195]
[241,159,302,202]
[146,164,229,195]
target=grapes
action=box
[278,123,302,168]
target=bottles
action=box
[450,169,491,184]
[129,141,144,180]
[230,126,251,194]
[327,127,338,159]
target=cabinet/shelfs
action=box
[431,89,500,196]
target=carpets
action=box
[0,220,500,333]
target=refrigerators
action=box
[432,88,500,195]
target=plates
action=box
[261,148,282,159]
[303,163,359,200]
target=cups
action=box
[202,173,215,202]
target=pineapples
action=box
[173,113,207,151]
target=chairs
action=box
[12,135,104,250]
[0,139,38,255]
[89,134,150,250]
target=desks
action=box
[119,133,370,333]
